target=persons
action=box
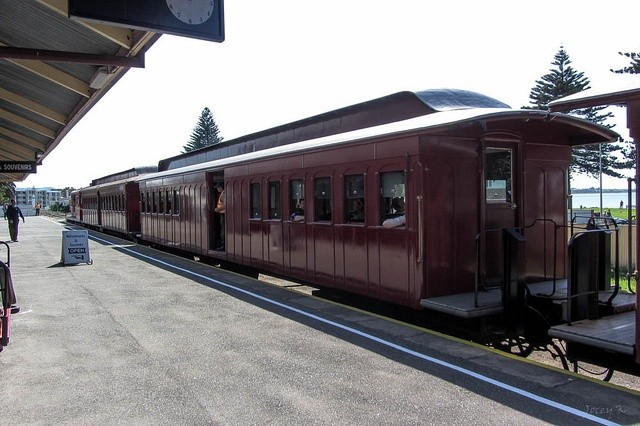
[391,197,405,213]
[214,182,225,250]
[293,198,305,222]
[6,198,25,243]
[350,200,363,219]
[381,204,406,228]
[35,203,41,216]
[3,203,9,220]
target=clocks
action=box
[166,0,214,26]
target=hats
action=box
[214,181,224,187]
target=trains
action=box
[68,80,640,381]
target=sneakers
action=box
[217,245,224,250]
